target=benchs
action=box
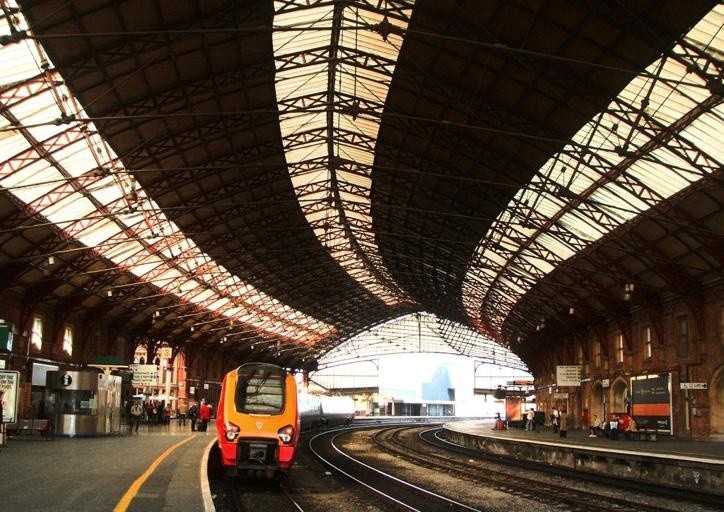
[628,430,657,441]
[14,419,55,441]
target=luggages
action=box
[609,429,618,440]
[197,421,206,431]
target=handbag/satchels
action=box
[610,421,618,429]
[189,408,194,417]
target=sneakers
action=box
[589,435,597,438]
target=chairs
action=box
[6,423,24,440]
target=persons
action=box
[126,400,213,432]
[552,407,638,440]
[524,408,535,431]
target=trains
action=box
[216,361,356,484]
